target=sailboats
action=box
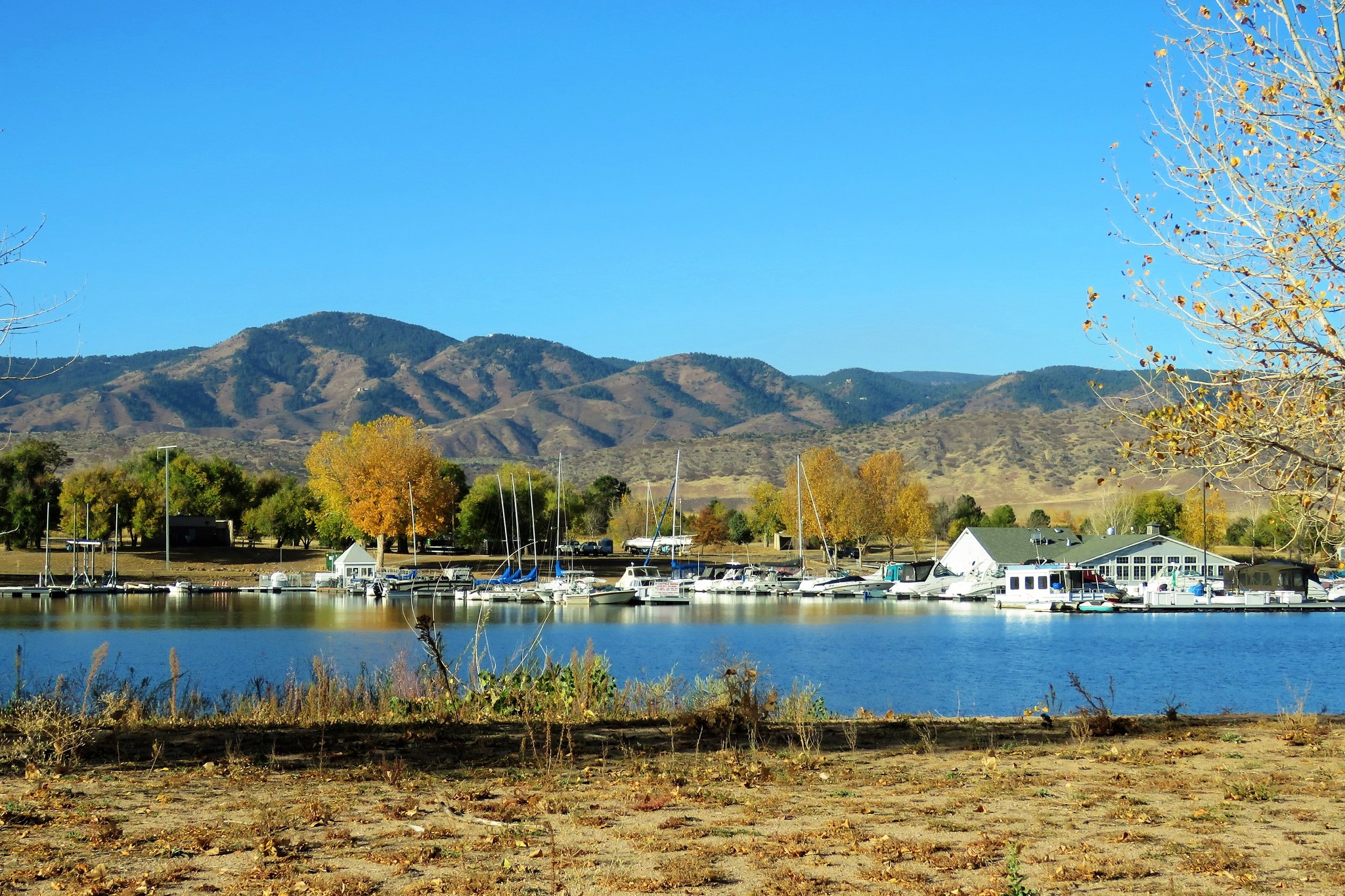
[622,479,698,552]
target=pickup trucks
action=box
[581,542,603,556]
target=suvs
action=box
[555,541,584,556]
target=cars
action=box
[826,547,859,560]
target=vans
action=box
[598,538,613,556]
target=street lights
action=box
[158,445,178,572]
[1202,448,1212,587]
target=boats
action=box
[350,447,1345,614]
[166,579,199,593]
